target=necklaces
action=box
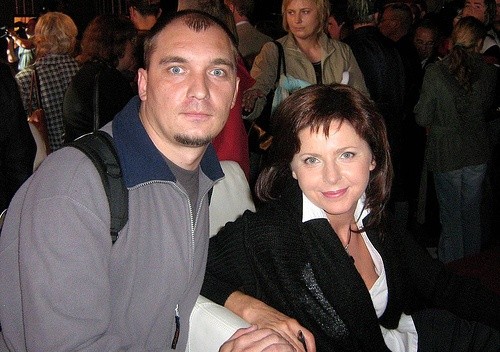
[344,226,352,253]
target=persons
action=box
[201,83,450,352]
[0,0,500,223]
[415,16,496,274]
[0,9,240,352]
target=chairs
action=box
[186,160,256,352]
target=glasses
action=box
[379,17,398,22]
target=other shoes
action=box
[383,201,396,218]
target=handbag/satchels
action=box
[27,69,51,157]
[248,121,274,189]
